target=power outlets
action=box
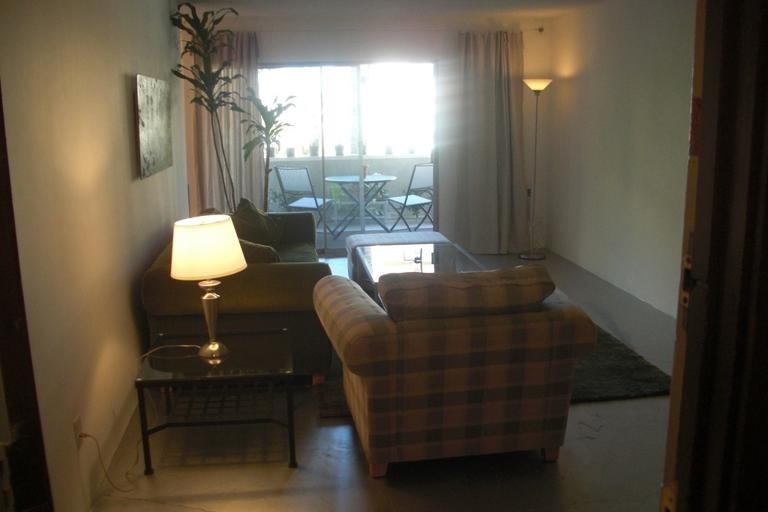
[71,419,89,445]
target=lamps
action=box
[170,214,247,358]
[519,77,553,260]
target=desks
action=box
[134,333,297,475]
[324,175,397,240]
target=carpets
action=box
[318,265,671,419]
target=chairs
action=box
[389,163,433,232]
[275,166,336,238]
[312,265,596,477]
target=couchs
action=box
[140,208,332,387]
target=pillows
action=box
[231,198,270,245]
[236,237,281,262]
